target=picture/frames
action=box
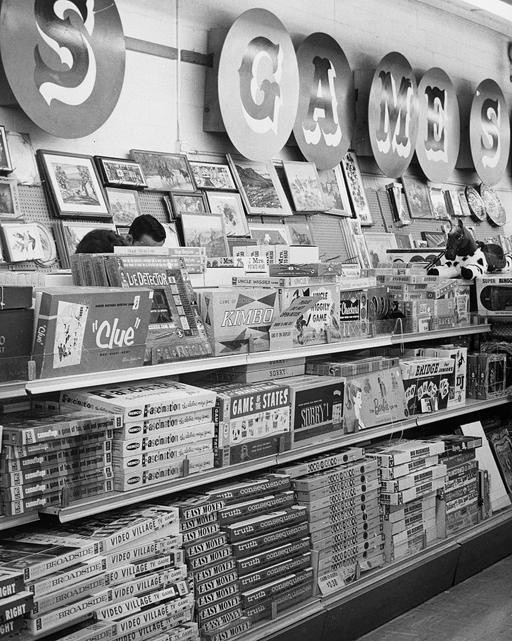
[0,124,450,268]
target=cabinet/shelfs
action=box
[0,323,511,641]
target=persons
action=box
[76,228,126,253]
[128,214,166,245]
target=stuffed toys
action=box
[430,219,507,280]
